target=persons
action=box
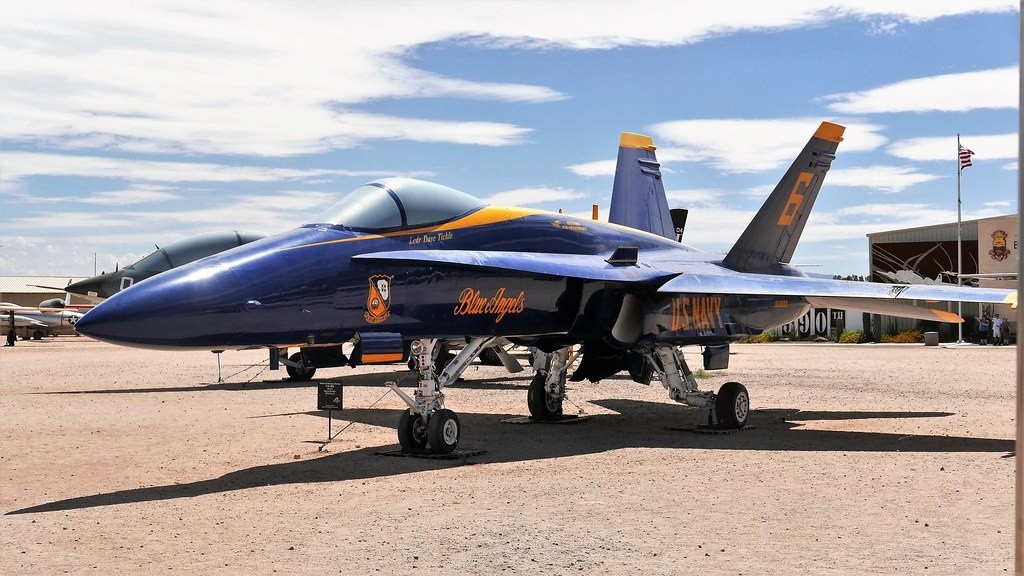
[975,314,1011,346]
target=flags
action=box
[959,144,975,171]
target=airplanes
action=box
[0,298,84,339]
[62,131,689,384]
[74,120,1021,458]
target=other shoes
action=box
[979,343,983,345]
[998,342,1001,345]
[984,343,986,345]
[993,343,997,345]
[1003,344,1009,345]
